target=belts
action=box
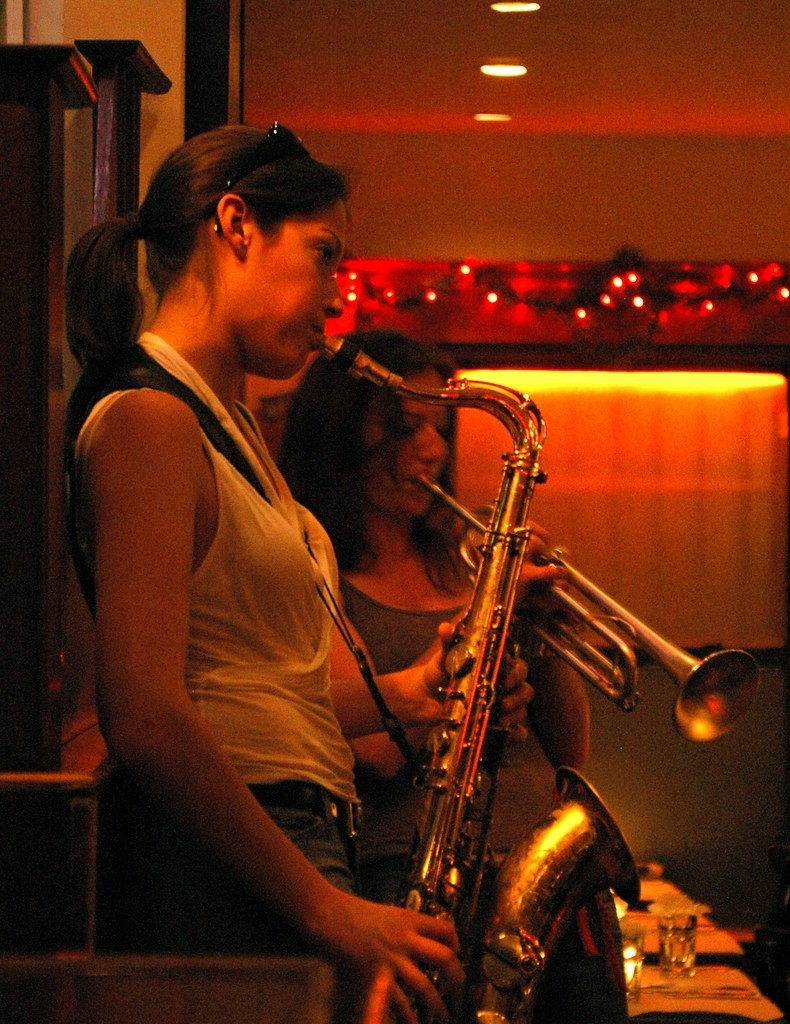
[249,782,365,841]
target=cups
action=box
[621,934,645,1004]
[658,913,698,980]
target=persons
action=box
[65,124,533,1024]
[274,329,627,1024]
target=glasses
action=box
[199,121,310,220]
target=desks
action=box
[609,865,785,1024]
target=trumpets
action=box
[413,471,759,744]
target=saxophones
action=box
[315,330,642,1024]
